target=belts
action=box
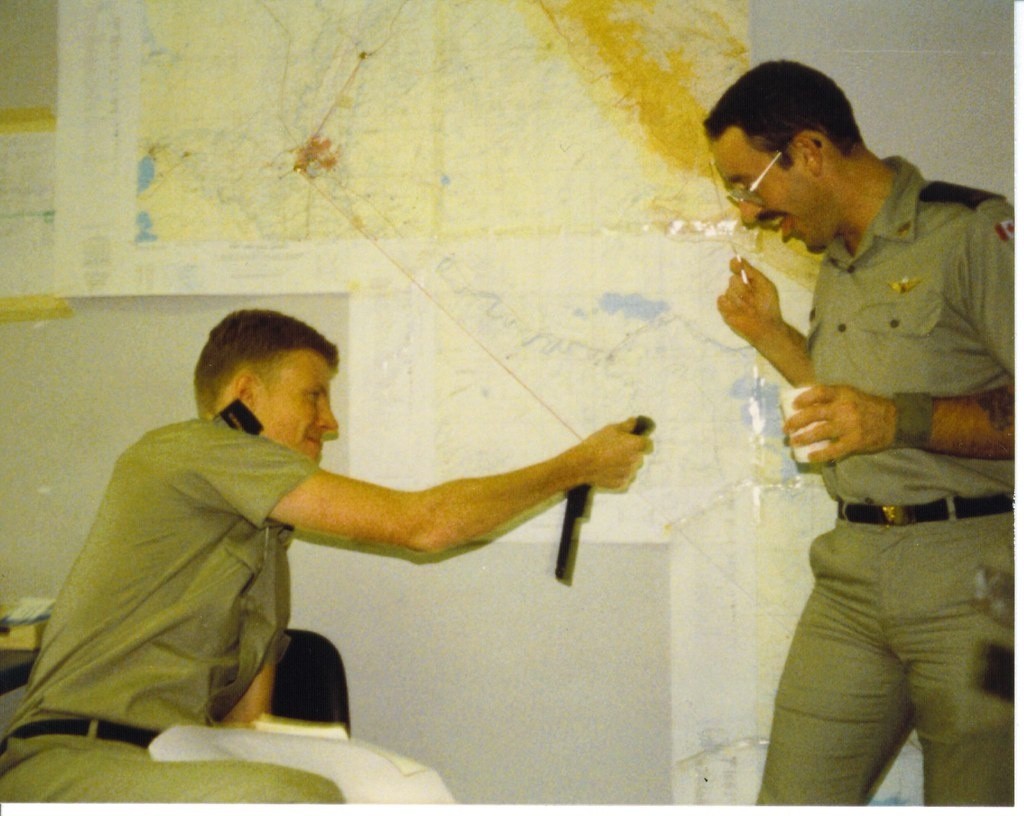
[837,494,1014,526]
[0,718,158,753]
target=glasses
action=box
[728,136,823,208]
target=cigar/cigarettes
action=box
[737,253,748,285]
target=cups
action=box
[779,386,832,463]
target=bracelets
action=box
[892,391,935,447]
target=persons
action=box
[703,59,1014,806]
[0,308,654,804]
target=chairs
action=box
[270,627,352,738]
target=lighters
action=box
[631,415,654,437]
